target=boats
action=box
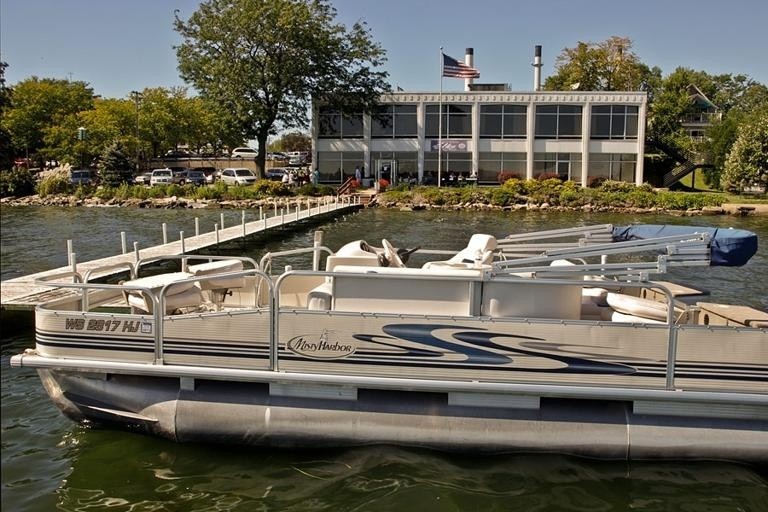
[6,225,767,467]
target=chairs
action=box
[421,233,498,273]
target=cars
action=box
[71,169,95,185]
[133,145,309,187]
[14,156,33,167]
[46,159,60,169]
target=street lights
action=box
[77,127,86,169]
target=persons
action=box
[281,165,479,190]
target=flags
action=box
[442,53,480,79]
